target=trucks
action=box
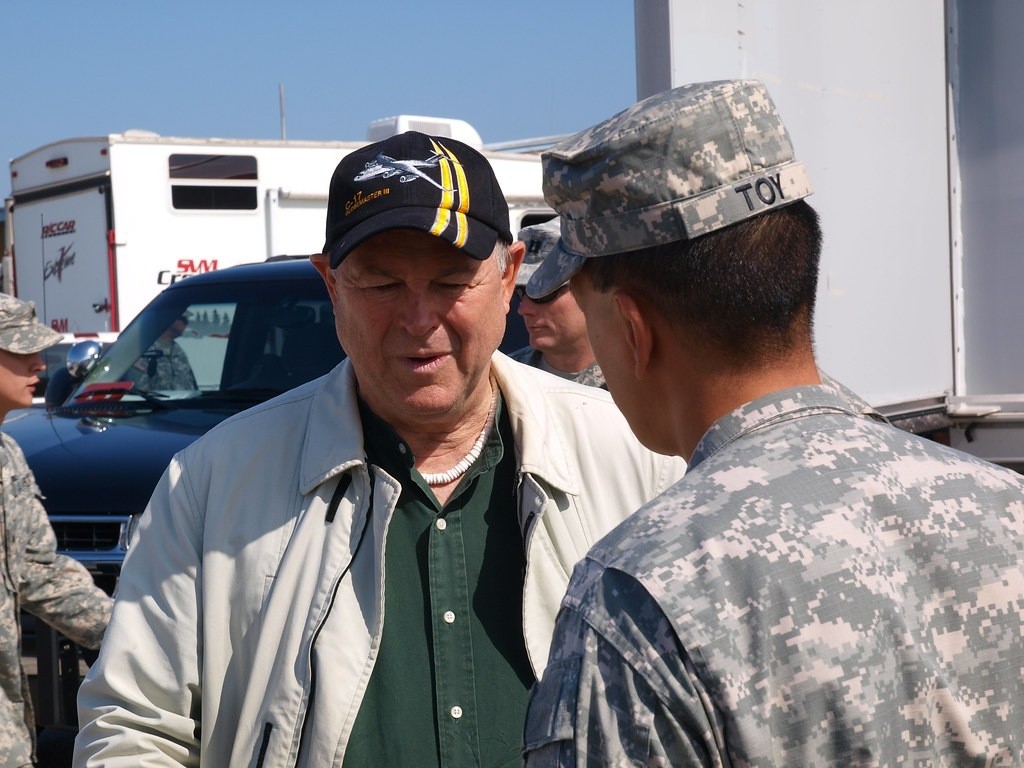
[9,116,562,338]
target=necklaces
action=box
[421,369,499,484]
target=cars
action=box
[0,253,545,586]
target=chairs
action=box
[230,306,312,393]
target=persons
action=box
[72,132,688,768]
[0,291,114,768]
[129,310,199,391]
[517,214,608,391]
[526,78,1024,768]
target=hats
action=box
[0,291,65,354]
[517,207,562,284]
[321,132,512,270]
[525,79,811,297]
[182,309,193,318]
